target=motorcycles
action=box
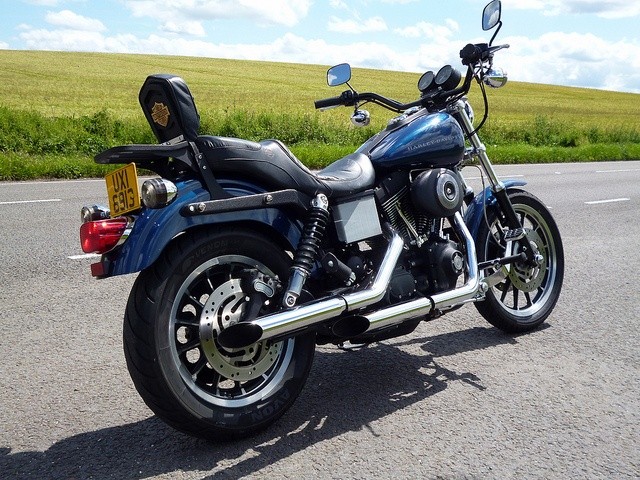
[81,0,564,441]
